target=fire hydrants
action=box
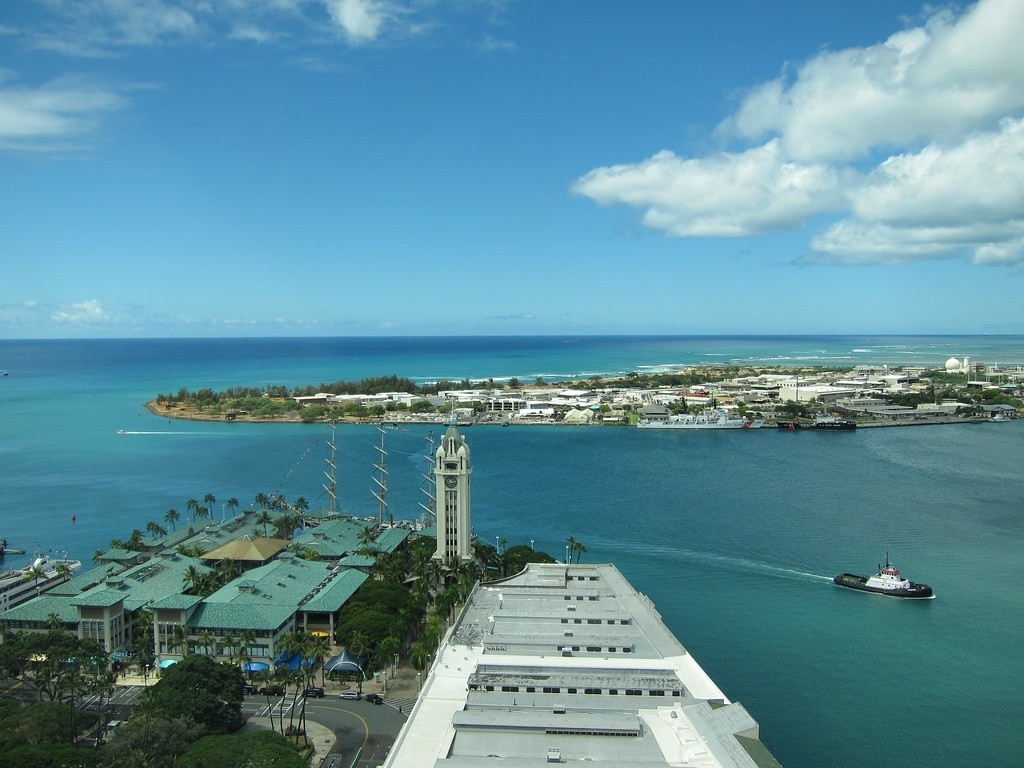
[399,706,402,713]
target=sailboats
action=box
[269,420,478,544]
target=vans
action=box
[339,692,363,701]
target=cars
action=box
[365,694,383,704]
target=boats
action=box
[636,410,767,429]
[834,549,932,597]
[988,413,1011,421]
[444,388,473,425]
[776,413,857,430]
[0,552,82,614]
[117,429,128,434]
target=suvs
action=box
[303,687,324,699]
[259,685,285,696]
[243,685,258,696]
